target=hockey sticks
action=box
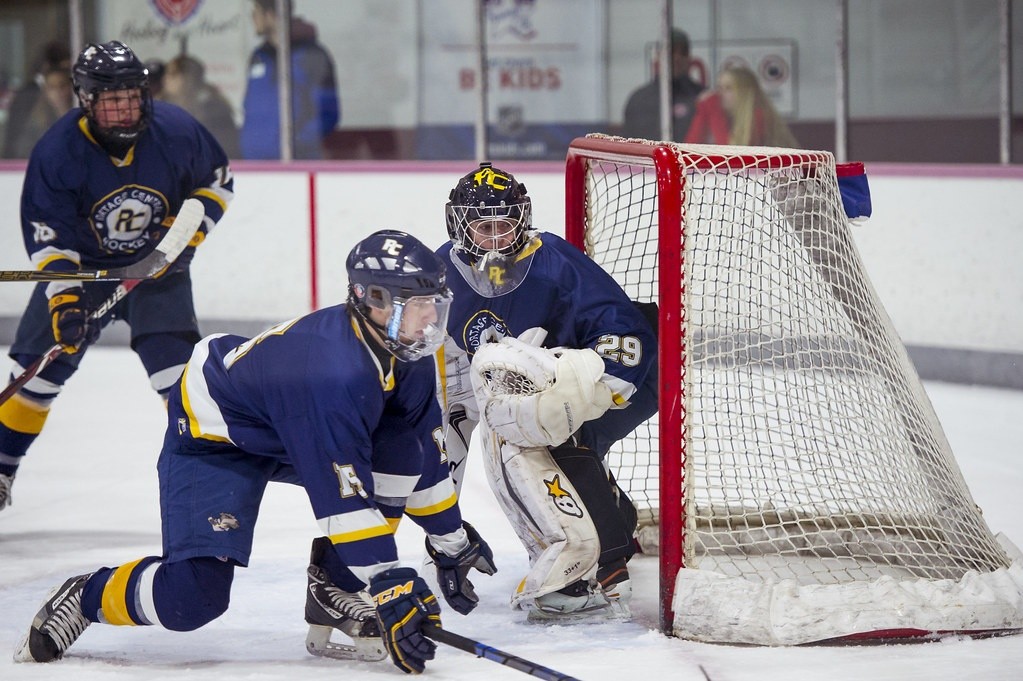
[0,197,206,283]
[416,622,581,681]
[0,280,141,406]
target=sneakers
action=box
[590,557,633,623]
[13,567,110,662]
[528,579,614,625]
[0,471,16,511]
[304,561,388,663]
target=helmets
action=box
[346,229,446,308]
[72,40,151,146]
[445,162,533,268]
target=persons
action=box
[14,229,497,674]
[0,38,234,508]
[238,0,339,158]
[427,162,658,618]
[622,30,707,142]
[685,68,800,148]
[146,56,238,158]
[0,44,73,157]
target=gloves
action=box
[369,567,442,673]
[123,237,196,290]
[49,286,102,355]
[424,520,497,615]
